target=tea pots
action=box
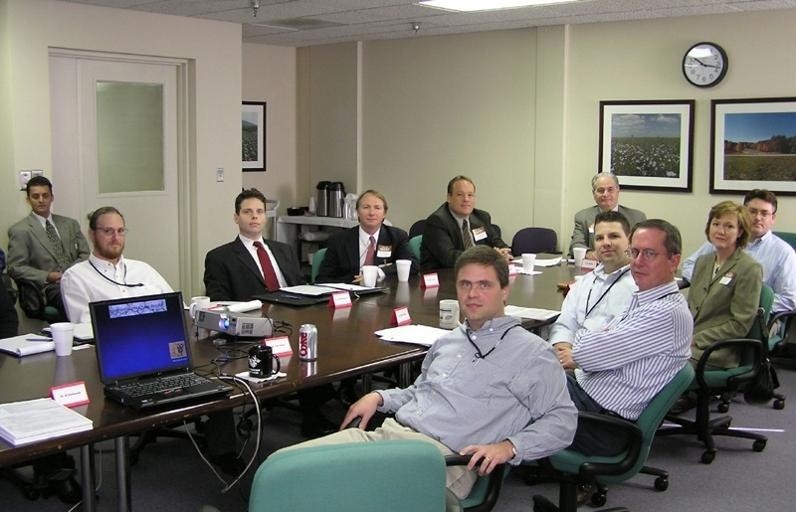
[317,181,347,218]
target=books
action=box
[0,396,94,447]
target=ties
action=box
[253,240,282,293]
[461,218,475,252]
[42,218,69,267]
[362,236,377,276]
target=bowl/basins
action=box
[286,207,309,216]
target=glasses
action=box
[627,247,671,259]
[94,226,129,237]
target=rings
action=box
[559,360,563,364]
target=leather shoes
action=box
[210,449,250,479]
[39,474,101,505]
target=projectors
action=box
[193,309,273,338]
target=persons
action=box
[569,171,647,262]
[548,211,639,370]
[0,248,83,505]
[59,206,246,476]
[314,189,420,408]
[7,175,90,318]
[267,244,580,512]
[680,189,796,339]
[557,219,695,456]
[420,176,514,272]
[686,201,763,374]
[204,188,340,440]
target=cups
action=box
[439,299,460,329]
[308,195,317,216]
[573,248,587,267]
[246,345,280,377]
[395,259,411,282]
[521,253,536,272]
[188,296,211,319]
[362,265,377,287]
[49,322,74,357]
[307,252,315,265]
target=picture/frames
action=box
[241,100,268,172]
[710,97,796,196]
[598,98,695,193]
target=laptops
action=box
[88,290,235,412]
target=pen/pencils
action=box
[281,294,299,299]
[25,339,53,341]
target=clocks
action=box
[681,41,728,88]
